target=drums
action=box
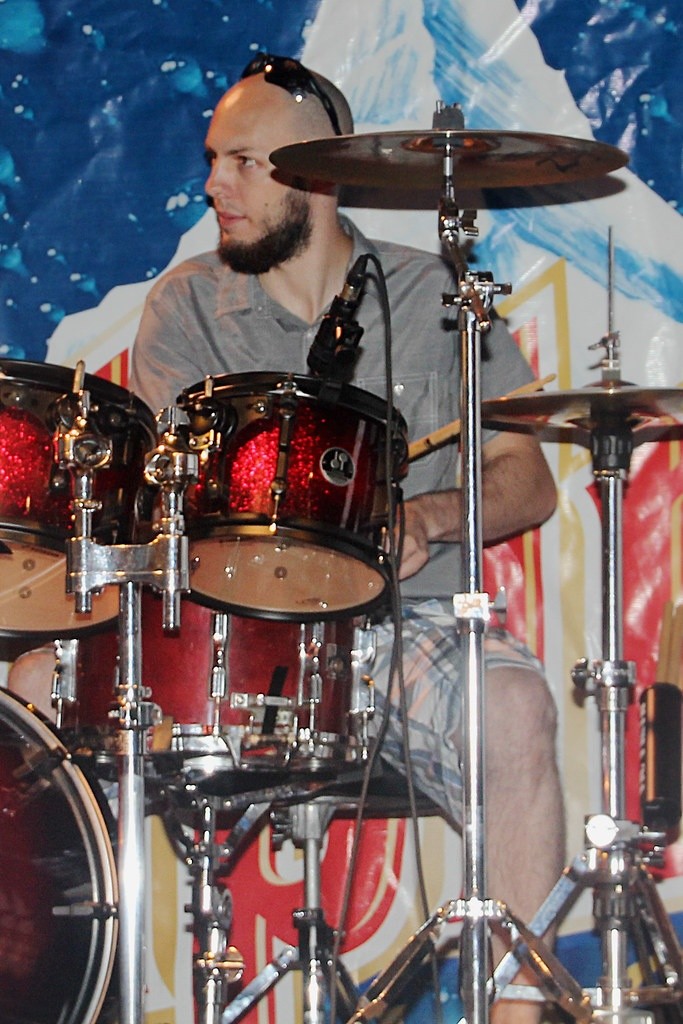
[153,370,409,624]
[0,687,122,1024]
[0,356,158,639]
[62,588,353,779]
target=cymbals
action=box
[268,128,629,192]
[481,379,683,431]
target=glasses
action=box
[241,53,342,136]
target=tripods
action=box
[220,156,682,1024]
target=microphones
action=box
[306,254,368,372]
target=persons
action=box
[7,69,566,1024]
